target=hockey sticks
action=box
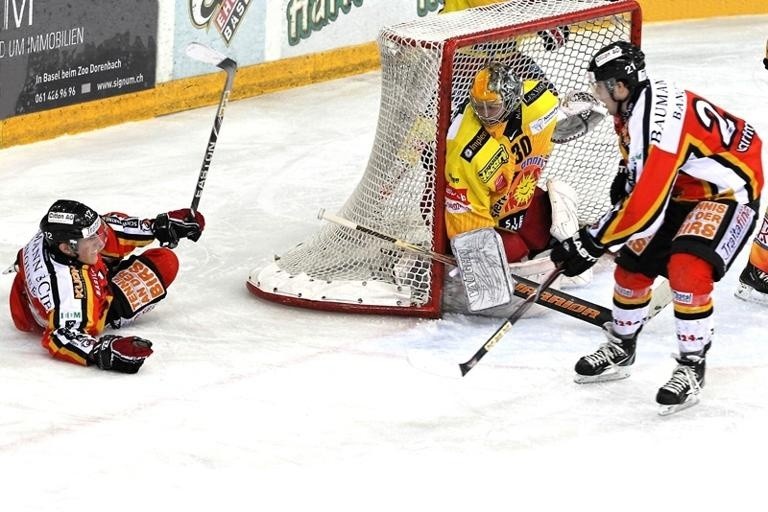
[406,259,565,378]
[318,208,674,332]
[184,43,237,212]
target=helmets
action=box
[38,198,102,268]
[588,41,651,100]
[469,60,527,126]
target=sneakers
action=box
[654,349,706,405]
[573,334,637,376]
[737,258,768,294]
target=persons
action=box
[7,198,204,376]
[365,62,609,325]
[549,40,764,405]
[736,203,767,298]
[372,0,568,208]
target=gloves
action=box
[549,222,607,277]
[609,155,632,207]
[152,208,206,248]
[94,334,154,376]
